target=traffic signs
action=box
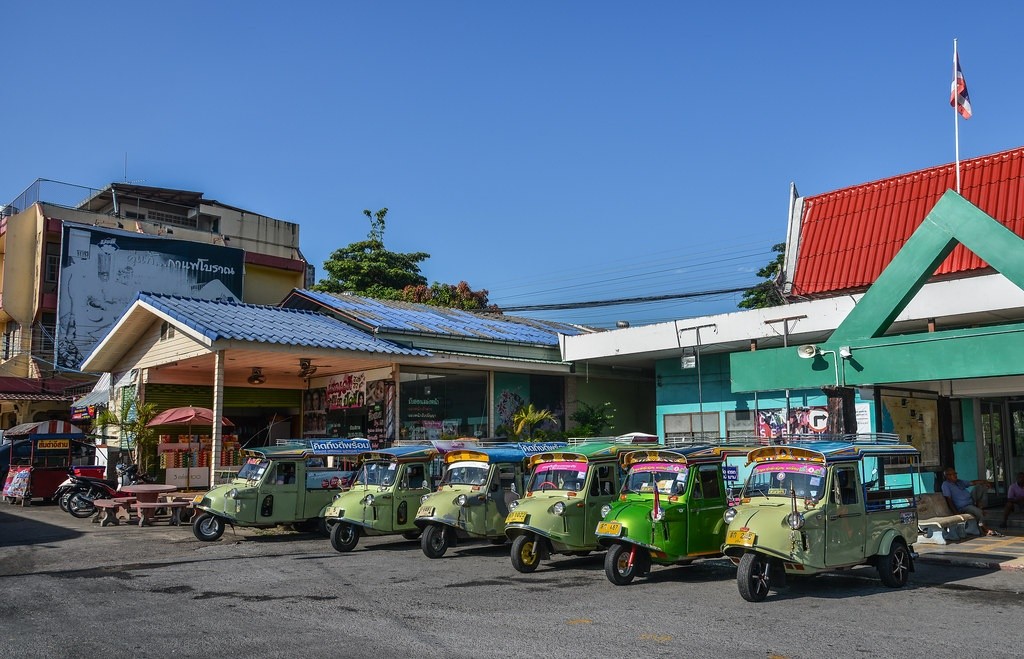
[808,407,830,433]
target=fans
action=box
[248,368,267,384]
[297,361,317,378]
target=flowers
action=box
[597,401,617,430]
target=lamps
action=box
[680,346,698,370]
[221,235,230,241]
[117,222,123,228]
[424,381,431,396]
[165,227,174,234]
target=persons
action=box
[941,467,994,537]
[282,463,295,484]
[312,388,320,410]
[304,412,326,431]
[358,390,364,406]
[999,472,1024,528]
[366,380,385,406]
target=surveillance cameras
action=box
[841,350,853,360]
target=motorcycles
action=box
[505,449,624,570]
[57,462,147,520]
[596,441,769,587]
[414,449,530,558]
[194,445,369,543]
[723,443,922,603]
[324,446,425,553]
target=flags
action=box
[950,51,973,120]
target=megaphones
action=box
[798,344,816,359]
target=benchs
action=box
[92,484,207,528]
[905,492,983,545]
